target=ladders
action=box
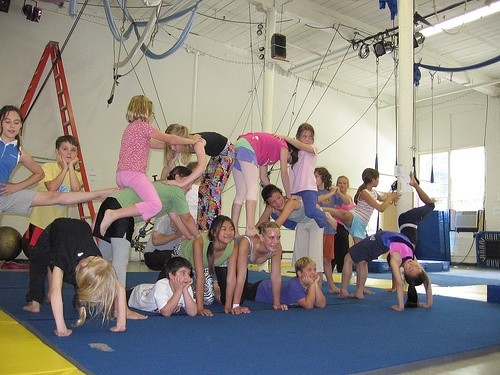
[20,39,102,247]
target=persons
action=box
[21,135,83,303]
[93,166,201,319]
[321,168,400,295]
[245,256,327,309]
[314,167,341,294]
[126,256,198,318]
[0,105,120,215]
[24,218,127,336]
[166,123,237,231]
[172,215,238,315]
[388,171,435,292]
[261,184,325,273]
[271,123,338,230]
[333,175,356,273]
[100,94,201,237]
[339,231,433,310]
[144,213,185,278]
[231,222,288,314]
[231,132,298,236]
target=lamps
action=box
[374,41,393,58]
[413,32,425,48]
[22,1,42,22]
